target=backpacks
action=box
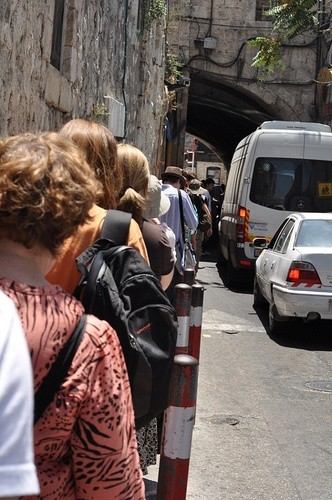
[73,209,180,431]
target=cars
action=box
[252,212,332,336]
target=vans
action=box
[218,120,332,287]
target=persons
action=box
[1,134,226,500]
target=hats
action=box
[189,179,201,189]
[142,174,171,219]
[162,166,188,184]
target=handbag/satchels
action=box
[199,215,211,233]
[184,241,197,272]
